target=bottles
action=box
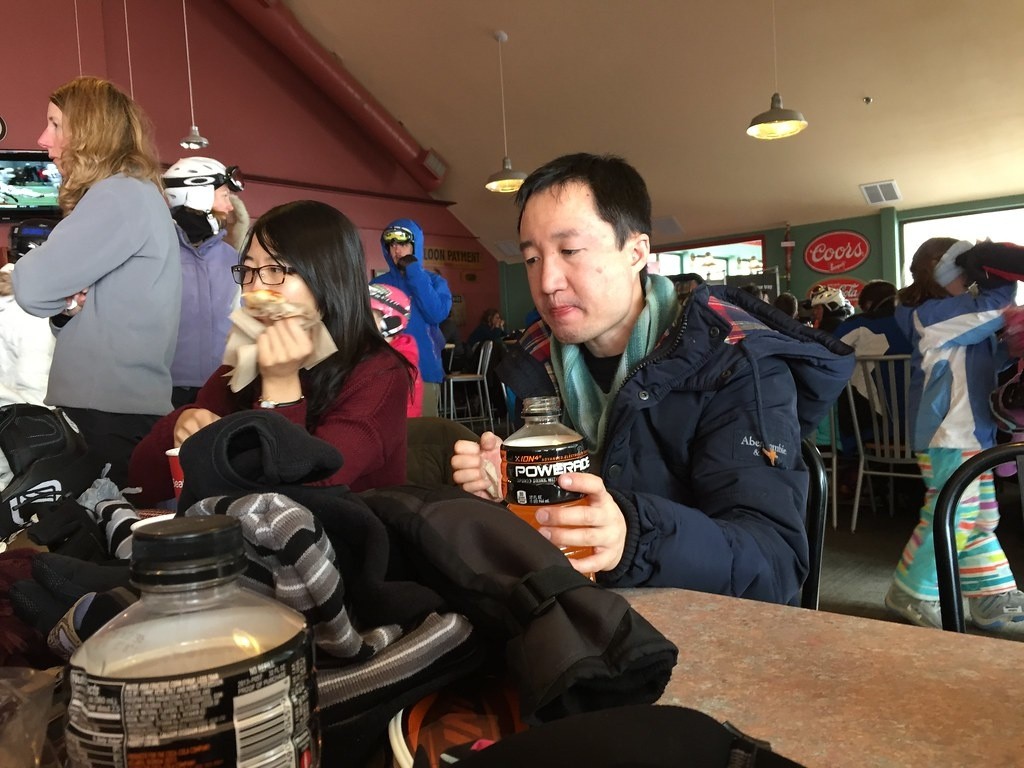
[500,397,596,583]
[64,518,322,767]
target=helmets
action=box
[161,156,228,213]
[366,283,411,337]
[7,218,56,265]
[812,287,846,311]
[382,224,416,244]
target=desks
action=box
[602,585,1024,768]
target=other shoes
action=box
[828,488,864,505]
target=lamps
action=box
[485,30,528,193]
[745,0,809,140]
[180,0,210,150]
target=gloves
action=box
[398,255,417,270]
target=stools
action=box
[444,340,495,435]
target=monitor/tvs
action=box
[0,149,63,218]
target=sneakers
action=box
[968,590,1024,634]
[887,581,943,630]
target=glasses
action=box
[227,166,246,192]
[231,263,297,285]
[809,285,826,299]
[384,229,412,243]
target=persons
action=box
[157,156,245,409]
[12,76,183,505]
[0,219,63,411]
[885,238,1024,631]
[451,153,857,605]
[729,281,934,495]
[367,283,423,417]
[462,310,507,418]
[369,218,452,418]
[126,201,410,524]
[160,156,250,411]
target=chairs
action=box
[802,353,1024,633]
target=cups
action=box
[165,447,184,500]
